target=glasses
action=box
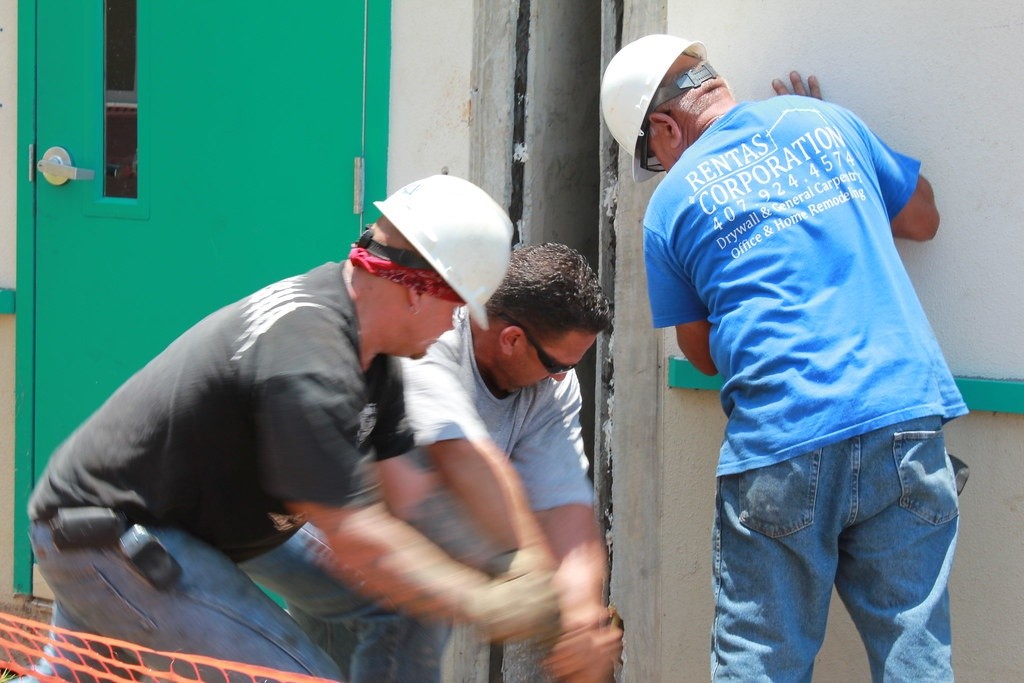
[640,111,671,172]
[496,310,578,374]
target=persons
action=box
[600,33,971,683]
[235,243,623,683]
[0,175,560,683]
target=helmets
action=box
[600,35,707,183]
[373,175,514,330]
